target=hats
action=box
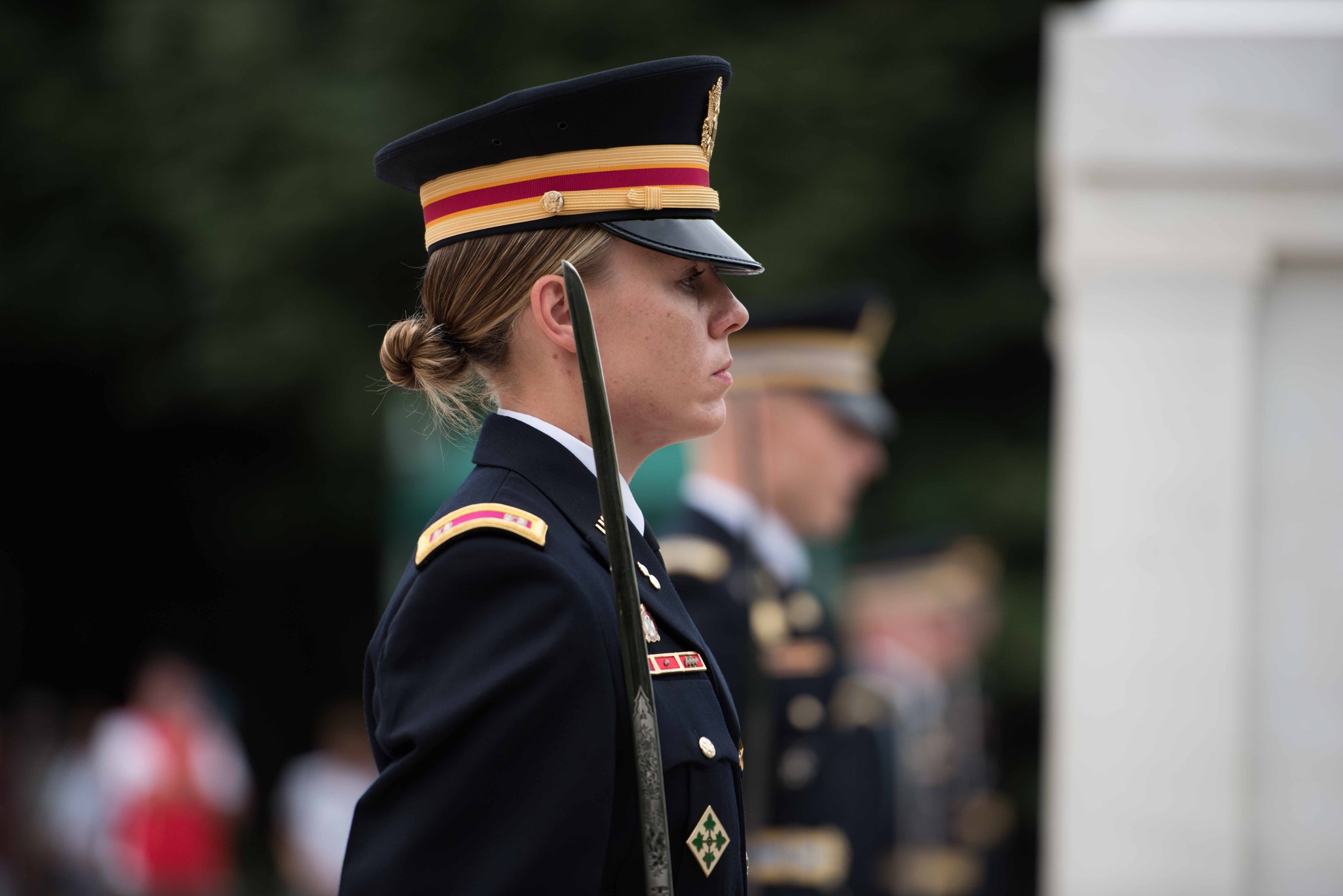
[720,289,898,434]
[373,57,765,277]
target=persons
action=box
[33,297,993,896]
[332,60,762,896]
